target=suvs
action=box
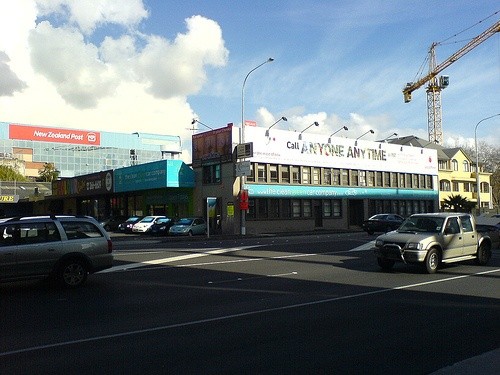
[0,214,114,289]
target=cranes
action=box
[402,10,500,145]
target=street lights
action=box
[474,113,500,207]
[240,58,274,236]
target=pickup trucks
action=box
[373,212,500,274]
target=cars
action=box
[99,215,129,231]
[118,216,145,233]
[169,218,207,236]
[362,213,406,235]
[150,217,179,236]
[132,216,166,234]
